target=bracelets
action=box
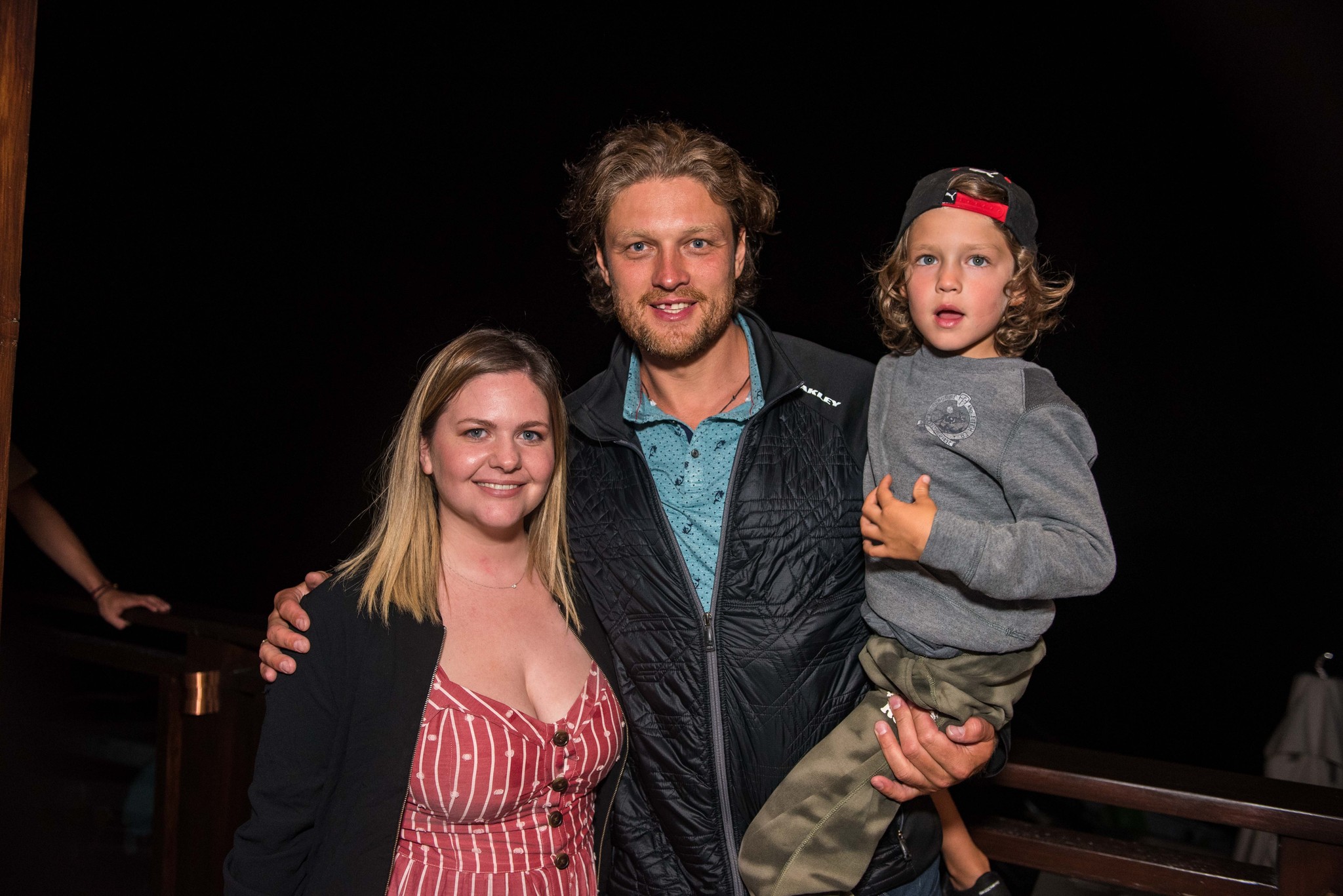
[91,582,116,599]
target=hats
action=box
[895,166,1038,258]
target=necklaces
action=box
[442,531,531,588]
[641,375,751,413]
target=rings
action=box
[260,639,268,647]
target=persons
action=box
[259,118,1012,896]
[8,447,171,630]
[740,166,1116,896]
[226,327,629,895]
